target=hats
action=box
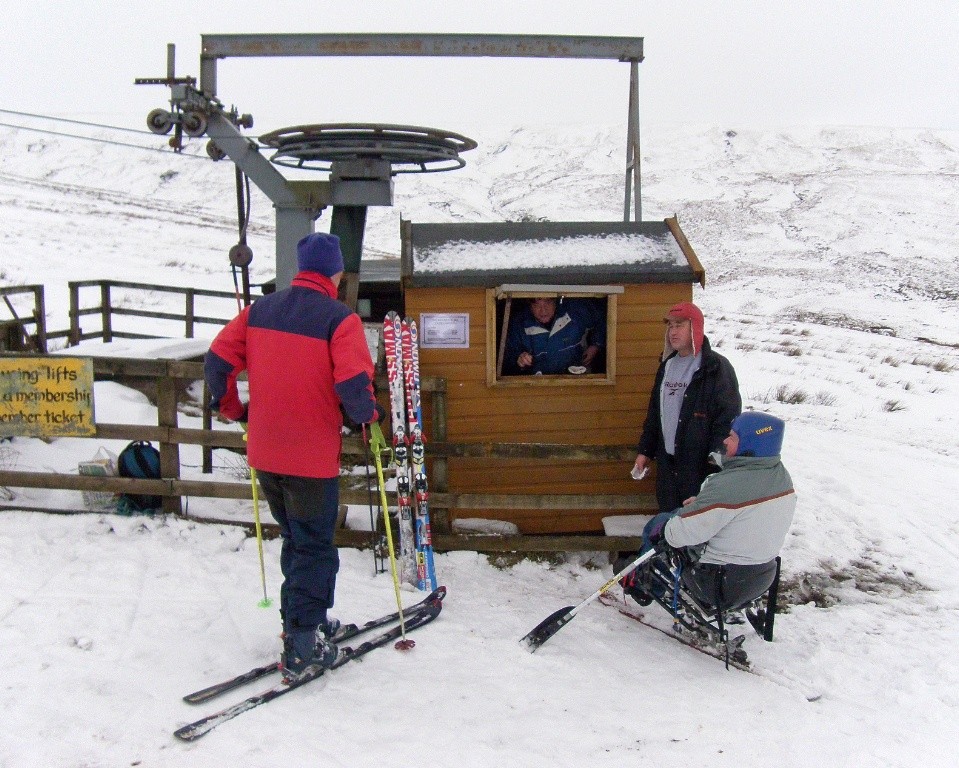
[661,302,704,362]
[297,232,344,277]
[730,412,785,456]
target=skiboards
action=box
[599,589,824,702]
[173,585,446,742]
[383,310,438,592]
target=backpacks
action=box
[117,439,163,508]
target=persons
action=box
[613,412,797,611]
[635,302,742,513]
[513,296,604,375]
[205,234,387,679]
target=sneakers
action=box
[613,558,652,606]
[319,617,359,643]
[282,643,338,683]
